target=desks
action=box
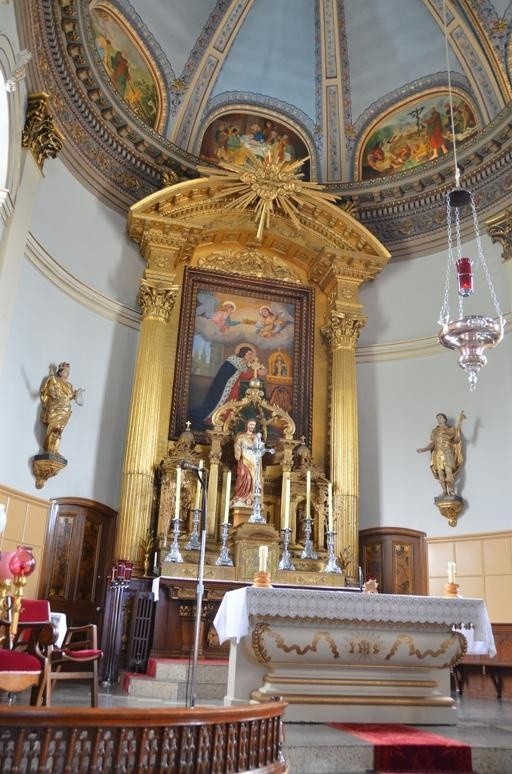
[212,586,498,725]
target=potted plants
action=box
[136,529,167,575]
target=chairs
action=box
[0,599,103,707]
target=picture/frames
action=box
[168,265,316,454]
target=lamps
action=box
[436,0,507,391]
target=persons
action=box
[232,416,266,508]
[244,305,286,336]
[207,300,242,332]
[416,410,467,497]
[39,362,87,454]
[187,342,270,426]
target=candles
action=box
[449,562,456,583]
[259,546,268,572]
[175,460,334,531]
